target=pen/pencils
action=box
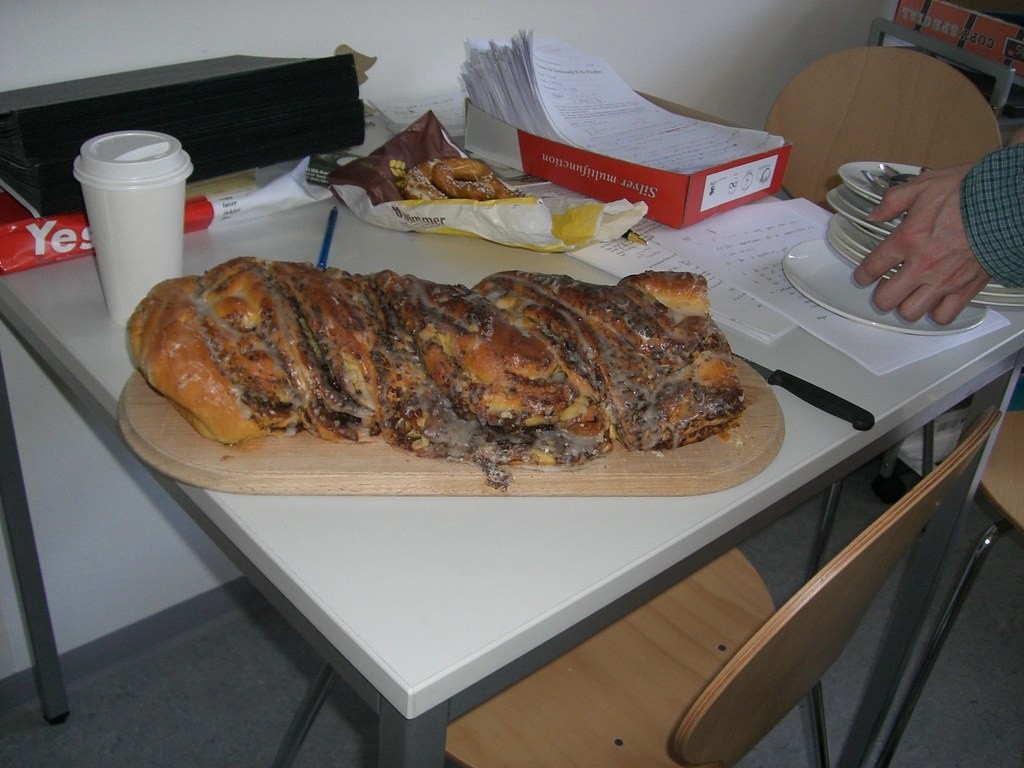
[316,206,338,268]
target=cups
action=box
[71,131,196,332]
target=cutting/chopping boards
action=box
[115,343,788,496]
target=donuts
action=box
[399,158,503,202]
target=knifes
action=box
[744,355,877,432]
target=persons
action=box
[853,125,1024,327]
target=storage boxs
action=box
[463,86,794,227]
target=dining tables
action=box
[1,86,1023,768]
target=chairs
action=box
[763,48,1005,218]
[269,405,1002,767]
[807,412,1022,767]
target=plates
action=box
[783,157,1024,337]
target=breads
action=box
[124,252,749,491]
